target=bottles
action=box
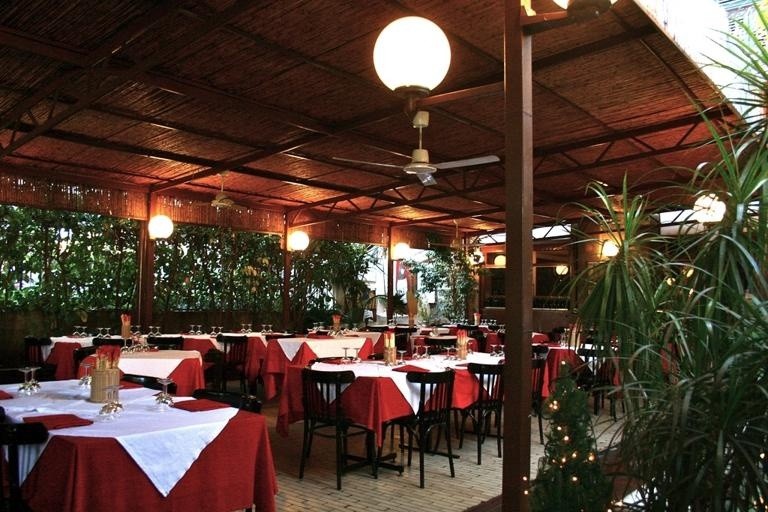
[312,322,359,338]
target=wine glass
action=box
[18,368,42,395]
[443,348,460,366]
[73,326,88,337]
[240,323,252,333]
[398,346,430,366]
[341,347,361,365]
[79,362,92,392]
[187,325,224,339]
[96,327,111,338]
[261,325,274,333]
[120,325,160,356]
[450,318,497,328]
[96,384,126,424]
[489,344,506,358]
[156,377,175,411]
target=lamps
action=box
[372,16,453,120]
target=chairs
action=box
[0,325,626,511]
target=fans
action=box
[193,170,248,213]
[331,110,500,188]
